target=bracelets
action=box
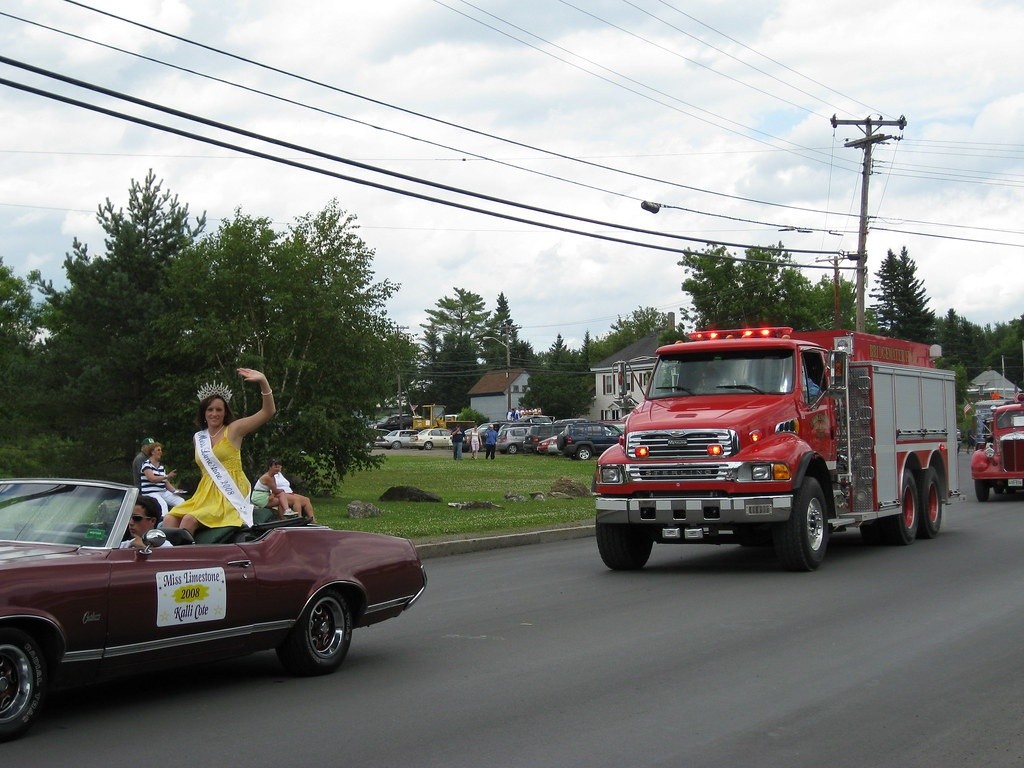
[261,388,273,395]
[164,475,168,479]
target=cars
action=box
[1,478,429,737]
[373,429,419,450]
[449,422,509,452]
[409,428,454,450]
[538,427,612,456]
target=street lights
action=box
[483,336,512,412]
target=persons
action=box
[771,361,823,396]
[467,424,482,459]
[115,496,174,547]
[140,442,185,519]
[998,416,1015,429]
[688,367,726,394]
[483,425,499,460]
[966,425,976,454]
[162,367,277,538]
[132,437,154,496]
[505,406,542,422]
[269,460,318,524]
[450,424,467,460]
[250,457,299,521]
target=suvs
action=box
[516,414,553,424]
[557,422,626,461]
[374,413,413,431]
[495,427,530,455]
[523,423,566,455]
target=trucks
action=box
[975,397,1013,449]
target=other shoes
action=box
[283,508,298,517]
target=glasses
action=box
[131,515,151,522]
[157,448,162,451]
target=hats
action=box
[490,425,493,428]
[142,438,154,444]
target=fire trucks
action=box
[589,327,959,570]
[970,395,1024,501]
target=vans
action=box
[497,422,539,432]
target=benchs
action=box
[196,508,279,545]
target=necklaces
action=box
[210,425,224,438]
